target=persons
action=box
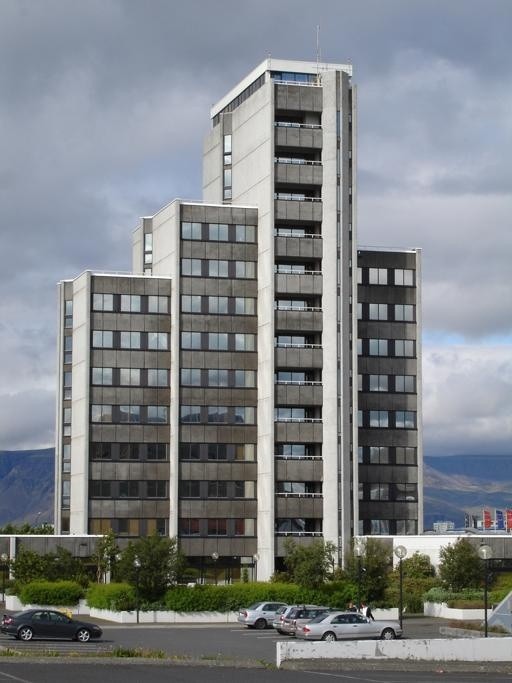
[345,602,357,612]
[360,601,371,618]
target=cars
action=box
[237,602,403,641]
[0,609,102,642]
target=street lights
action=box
[478,546,493,638]
[212,552,219,586]
[396,545,407,629]
[353,545,364,612]
[1,553,7,602]
[134,559,142,624]
[253,554,261,583]
